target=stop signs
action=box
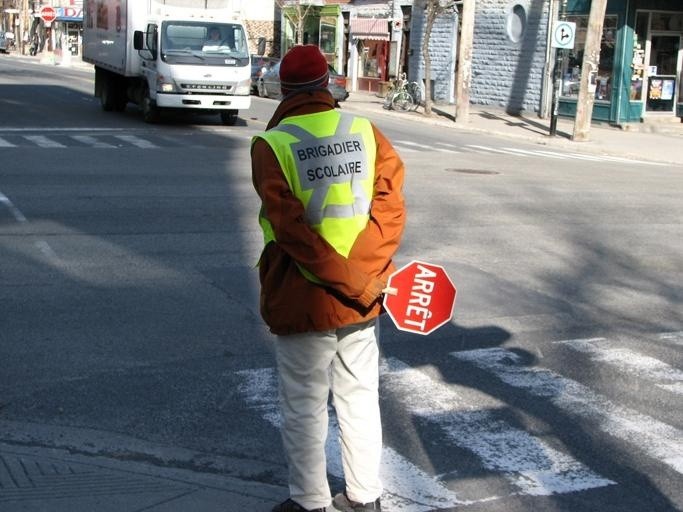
[40,7,56,22]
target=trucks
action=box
[81,0,252,125]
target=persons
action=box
[248,44,406,512]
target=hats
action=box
[279,44,330,97]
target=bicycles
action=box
[384,72,421,114]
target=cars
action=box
[248,53,281,95]
[553,43,614,75]
[0,31,8,53]
[257,58,348,105]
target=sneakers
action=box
[271,498,326,512]
[331,487,381,512]
